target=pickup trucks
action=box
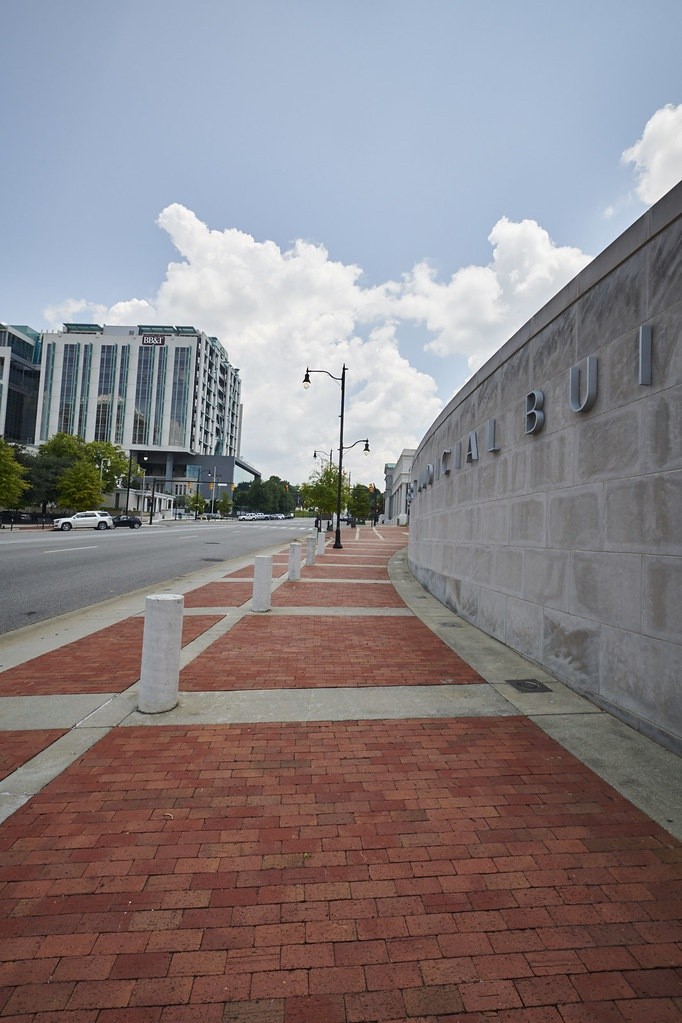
[256,513,269,520]
[238,513,256,521]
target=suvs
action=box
[112,515,142,529]
[51,510,113,532]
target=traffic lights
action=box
[195,470,211,520]
[231,486,234,491]
[369,484,374,492]
[286,485,288,493]
[188,482,192,489]
[210,483,213,490]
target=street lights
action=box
[125,454,149,516]
[302,366,370,550]
[95,458,111,510]
[313,450,332,473]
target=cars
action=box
[278,514,284,520]
[0,511,15,523]
[270,515,278,520]
[15,511,32,523]
